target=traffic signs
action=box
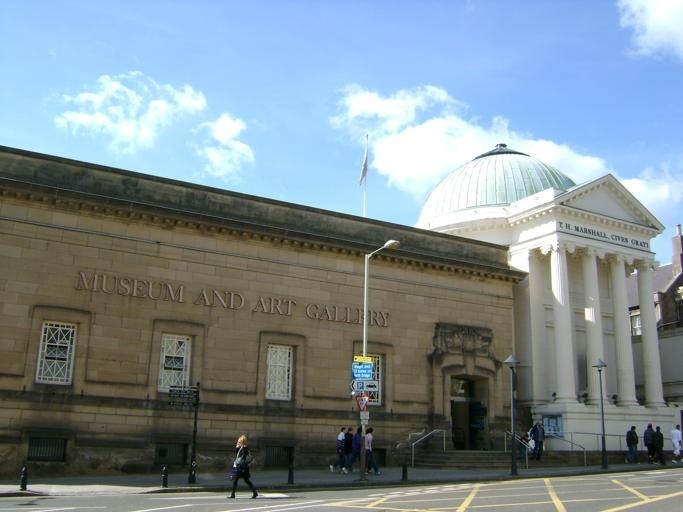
[167,384,198,408]
[347,380,379,393]
[352,355,374,363]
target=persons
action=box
[644,424,656,464]
[652,426,668,466]
[518,420,545,461]
[226,434,259,499]
[670,424,683,465]
[624,426,642,465]
[329,428,382,475]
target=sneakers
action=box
[625,456,683,465]
[252,493,258,498]
[329,464,383,476]
[227,495,236,498]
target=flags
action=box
[357,142,368,187]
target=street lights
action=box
[589,356,609,471]
[501,353,521,478]
[353,236,400,485]
